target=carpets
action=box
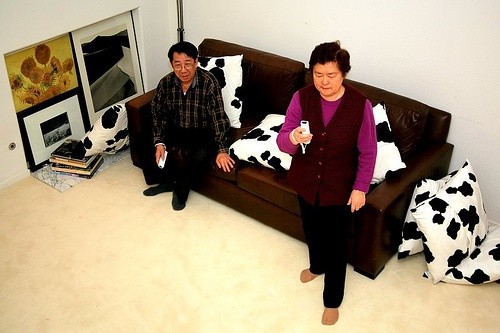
[30,150,129,193]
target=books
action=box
[154,142,167,148]
[48,138,104,179]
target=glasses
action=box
[172,62,196,71]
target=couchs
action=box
[124,38,455,280]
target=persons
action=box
[142,41,235,211]
[276,42,377,325]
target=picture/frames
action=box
[4,32,82,114]
[16,88,92,172]
[70,9,145,131]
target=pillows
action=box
[398,171,459,260]
[71,105,128,158]
[368,102,408,190]
[229,113,293,171]
[422,225,500,285]
[412,159,491,285]
[198,54,244,128]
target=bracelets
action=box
[291,128,299,143]
